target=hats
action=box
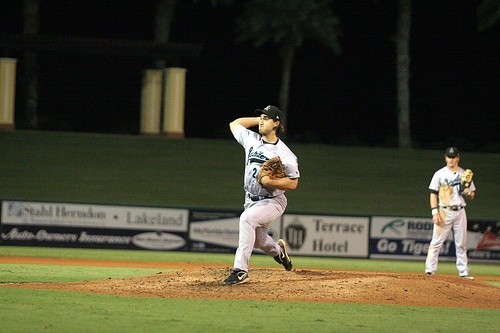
[445,146,459,157]
[256,105,283,122]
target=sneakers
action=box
[222,268,250,285]
[274,239,292,271]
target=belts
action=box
[443,206,463,211]
[248,193,268,201]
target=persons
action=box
[223,106,300,284]
[425,147,476,279]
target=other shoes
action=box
[460,274,473,279]
[426,272,432,276]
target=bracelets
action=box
[431,207,438,215]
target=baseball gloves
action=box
[460,168,473,193]
[257,155,284,188]
